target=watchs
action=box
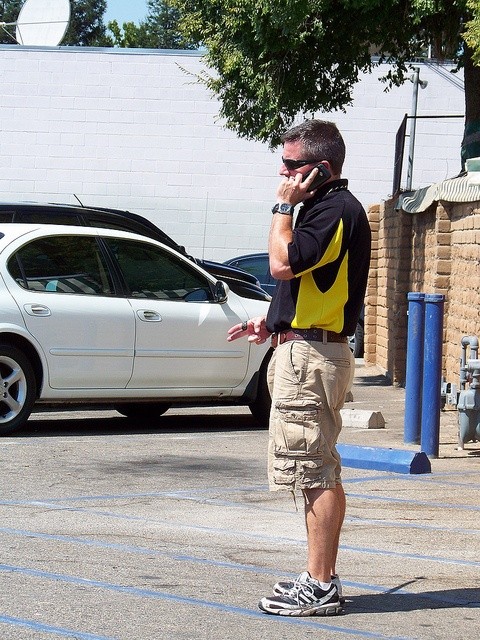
[271,200,294,217]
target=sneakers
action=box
[273,572,343,604]
[258,571,342,617]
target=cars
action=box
[0,193,260,286]
[223,254,362,356]
[0,224,274,428]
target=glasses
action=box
[282,156,328,170]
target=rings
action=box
[241,320,249,332]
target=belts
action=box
[271,329,348,348]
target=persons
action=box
[226,119,375,621]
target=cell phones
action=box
[302,164,331,193]
